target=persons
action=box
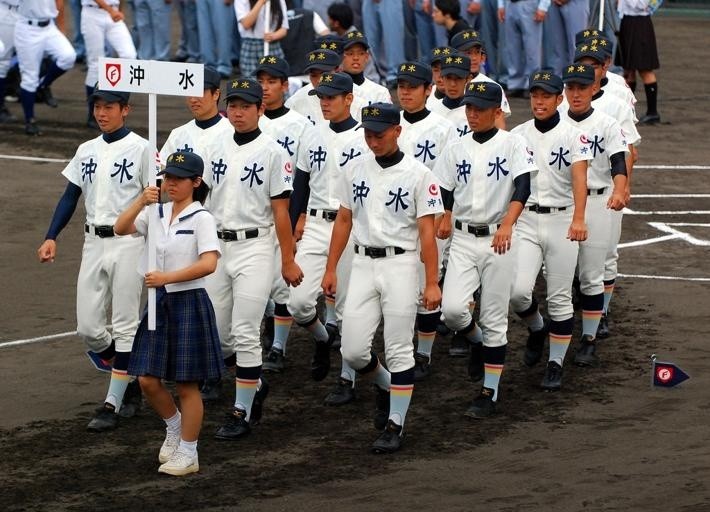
[615,2,659,125]
[0,1,139,127]
[114,151,222,477]
[38,27,395,432]
[197,30,512,442]
[137,1,620,90]
[320,30,631,455]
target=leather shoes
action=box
[504,88,523,99]
[523,88,530,100]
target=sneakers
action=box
[639,112,661,125]
[259,345,286,375]
[467,340,486,384]
[199,376,225,403]
[212,406,251,442]
[572,286,583,312]
[0,105,18,124]
[87,117,102,130]
[573,334,597,367]
[523,317,550,367]
[85,400,122,433]
[539,359,565,391]
[411,352,433,381]
[322,322,343,351]
[261,316,276,352]
[248,377,271,426]
[310,324,336,383]
[372,382,393,433]
[597,313,609,339]
[37,87,60,108]
[25,122,42,136]
[369,418,406,455]
[159,425,184,464]
[116,393,144,420]
[448,331,471,359]
[462,386,504,419]
[157,449,200,477]
[323,376,359,408]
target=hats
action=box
[342,31,370,50]
[314,35,344,55]
[575,29,606,47]
[355,102,400,133]
[429,46,459,67]
[304,49,341,73]
[222,80,263,104]
[156,150,206,180]
[450,30,483,51]
[584,37,614,57]
[205,64,222,91]
[529,70,563,95]
[574,43,607,63]
[308,72,353,97]
[439,53,472,79]
[88,79,132,106]
[251,55,290,78]
[394,62,433,87]
[563,64,596,85]
[459,82,503,109]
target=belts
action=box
[310,209,337,221]
[218,229,257,240]
[354,244,406,259]
[455,220,502,238]
[84,224,115,238]
[529,206,567,214]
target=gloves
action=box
[587,189,604,195]
